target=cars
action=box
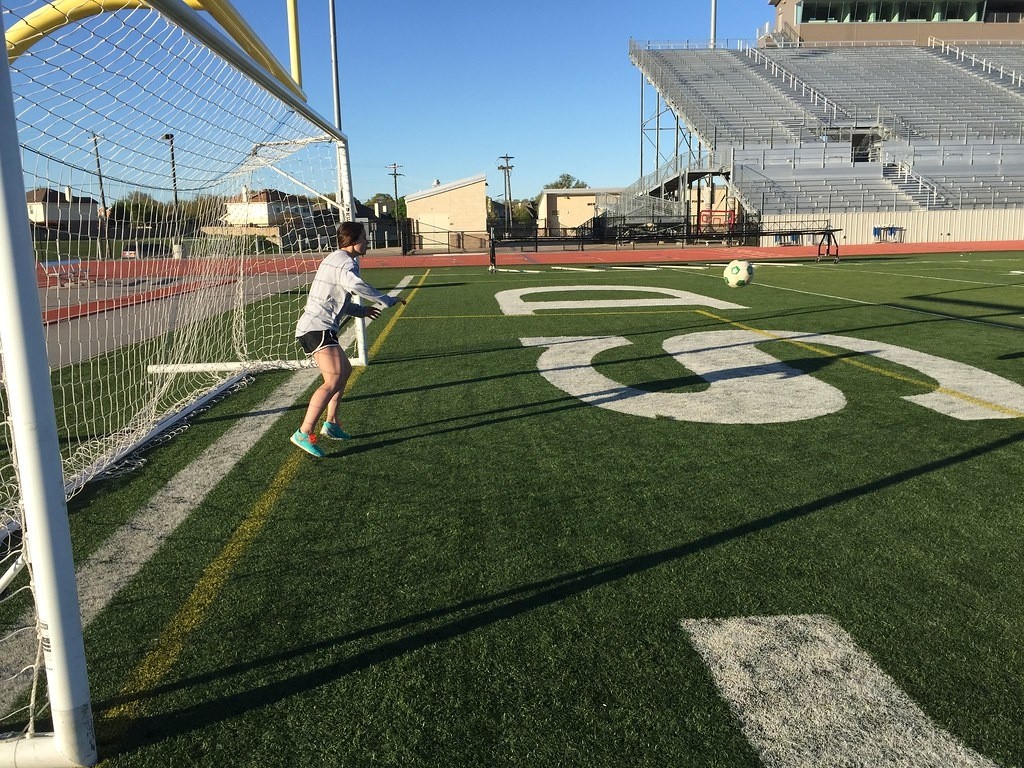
[121,242,173,259]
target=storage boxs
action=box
[411,233,489,249]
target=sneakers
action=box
[290,429,325,457]
[320,422,351,441]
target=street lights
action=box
[161,133,183,246]
[496,166,513,239]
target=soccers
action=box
[723,259,753,288]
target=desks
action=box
[874,227,907,243]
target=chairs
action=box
[40,259,90,287]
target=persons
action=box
[290,221,407,458]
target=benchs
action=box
[695,239,737,246]
[633,45,1024,216]
